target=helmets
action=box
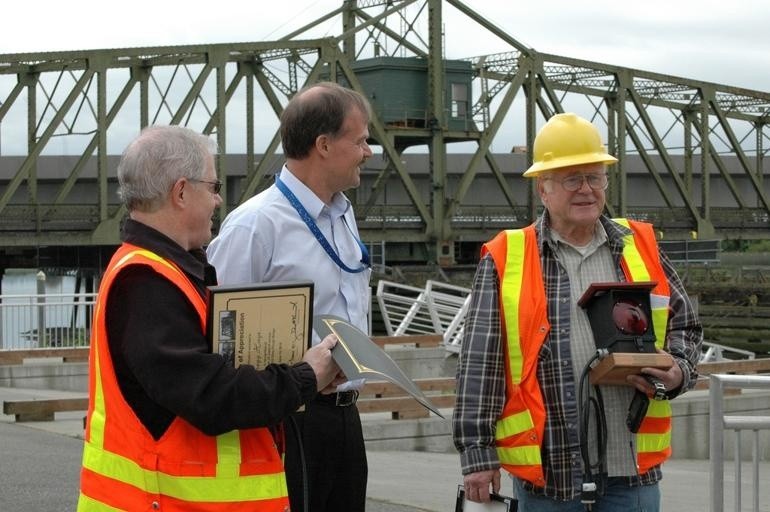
[521,112,620,179]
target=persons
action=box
[70,120,352,510]
[448,111,706,512]
[198,79,371,510]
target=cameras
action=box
[625,390,650,433]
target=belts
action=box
[332,390,358,407]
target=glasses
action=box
[187,179,224,195]
[559,172,611,192]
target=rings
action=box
[652,382,667,401]
